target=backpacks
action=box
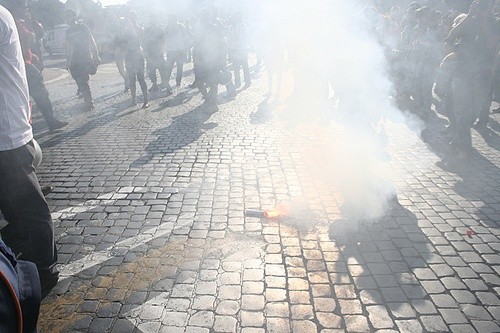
[0,234,42,332]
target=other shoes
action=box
[49,120,68,130]
[492,108,500,113]
[76,91,83,99]
[86,100,93,110]
[40,186,55,196]
[124,81,253,114]
[184,59,191,63]
[474,122,486,132]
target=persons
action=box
[1,0,500,333]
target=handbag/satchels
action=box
[89,60,98,75]
